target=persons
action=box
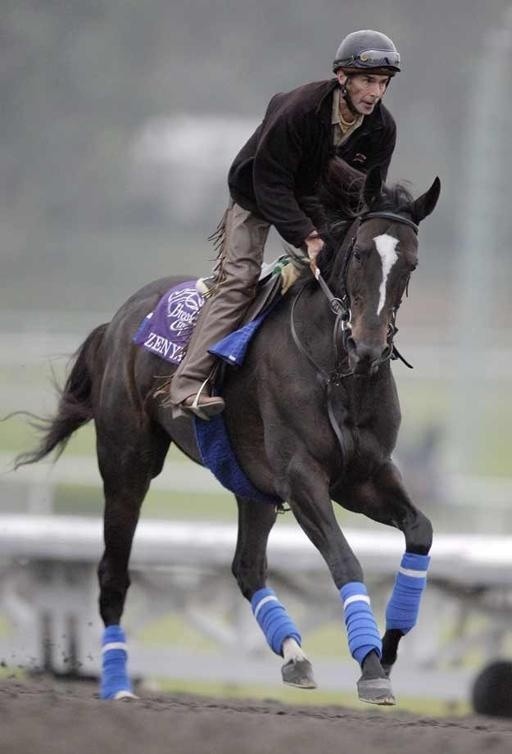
[169,28,397,428]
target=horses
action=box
[0,164,441,707]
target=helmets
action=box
[333,30,399,73]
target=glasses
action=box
[358,49,399,69]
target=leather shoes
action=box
[171,393,224,422]
